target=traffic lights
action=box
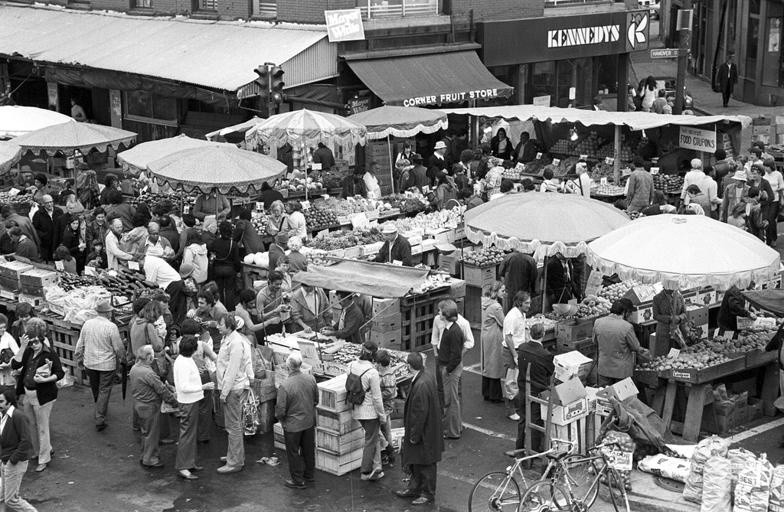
[270,66,284,108]
[252,63,269,110]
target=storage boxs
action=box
[314,426,366,456]
[314,448,364,476]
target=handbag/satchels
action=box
[160,392,180,413]
[242,401,261,436]
[36,359,74,387]
[153,350,168,377]
[1,348,14,364]
[680,319,703,345]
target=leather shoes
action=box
[412,497,432,506]
[178,470,199,480]
[192,464,204,471]
[394,489,419,496]
[286,478,306,489]
[140,455,164,469]
[160,438,176,444]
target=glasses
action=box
[28,339,41,346]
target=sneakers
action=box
[360,444,394,481]
[217,464,241,474]
[509,414,520,420]
[220,454,228,462]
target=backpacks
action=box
[345,364,371,406]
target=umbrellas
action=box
[12,123,138,156]
[0,105,73,142]
[244,108,365,200]
[203,116,265,138]
[345,105,448,195]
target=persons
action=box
[170,335,205,478]
[0,141,363,436]
[363,126,596,217]
[512,322,553,460]
[128,343,177,469]
[625,136,783,247]
[431,299,475,440]
[0,388,40,512]
[346,341,384,482]
[718,53,738,107]
[624,75,695,116]
[395,350,444,504]
[215,313,248,475]
[272,353,318,488]
[480,249,760,421]
[11,327,67,471]
[69,96,86,122]
[372,222,417,264]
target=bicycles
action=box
[467,435,599,511]
[516,439,632,511]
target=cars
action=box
[637,76,694,110]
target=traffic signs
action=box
[648,48,679,59]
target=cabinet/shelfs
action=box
[0,127,783,443]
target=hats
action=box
[383,225,397,233]
[181,213,196,227]
[275,230,289,244]
[731,170,747,182]
[404,352,423,370]
[752,164,766,177]
[92,207,108,217]
[411,153,424,162]
[95,300,113,312]
[435,140,448,151]
